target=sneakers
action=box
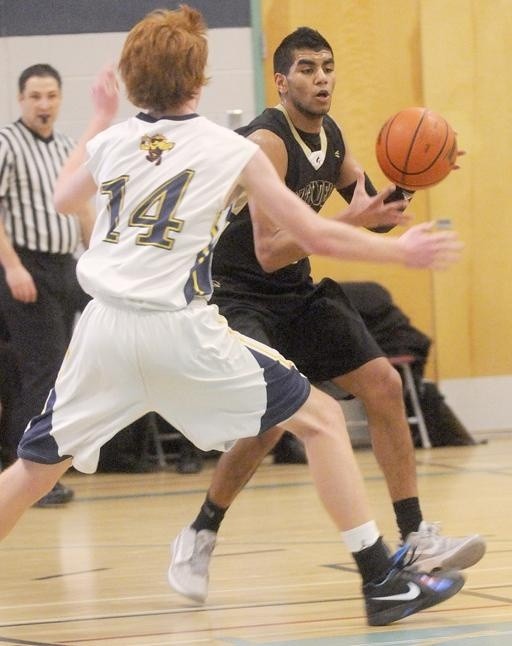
[32,482,74,508]
[401,521,485,577]
[362,545,464,626]
[168,518,218,603]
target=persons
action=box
[0,3,465,626]
[0,63,97,508]
[168,25,487,602]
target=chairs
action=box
[345,355,430,451]
[142,413,184,470]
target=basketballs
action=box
[374,107,459,192]
